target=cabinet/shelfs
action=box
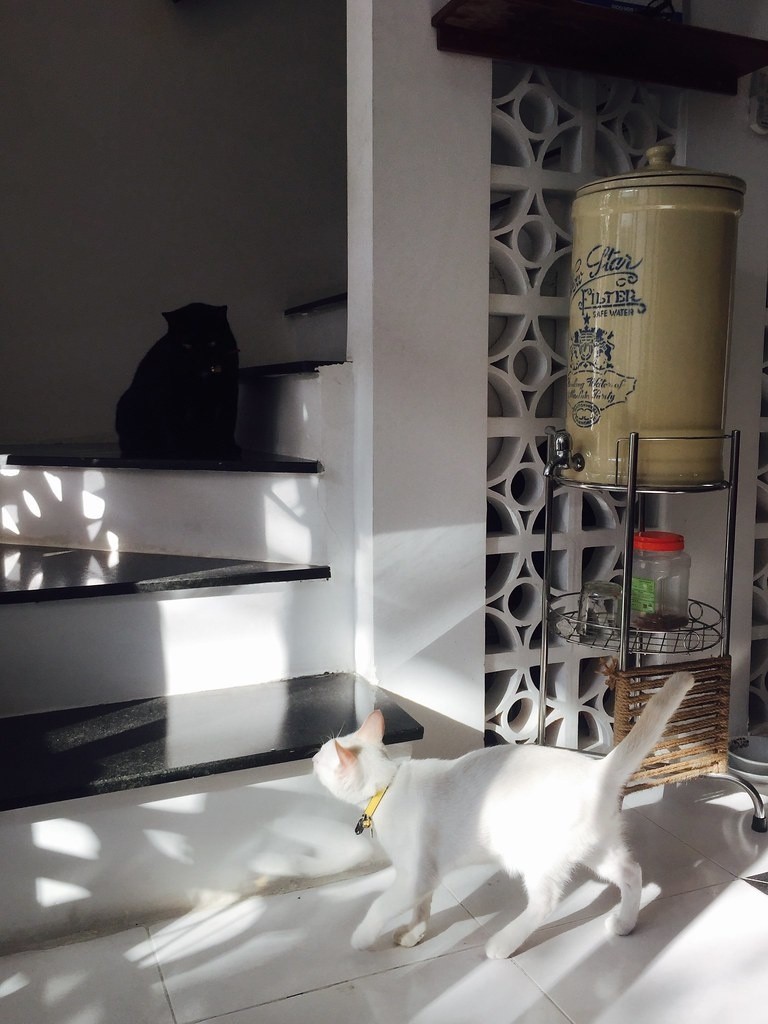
[537,426,768,836]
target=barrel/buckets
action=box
[562,145,747,486]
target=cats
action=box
[313,672,697,961]
[115,302,246,463]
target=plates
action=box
[727,736,768,784]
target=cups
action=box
[577,582,624,639]
